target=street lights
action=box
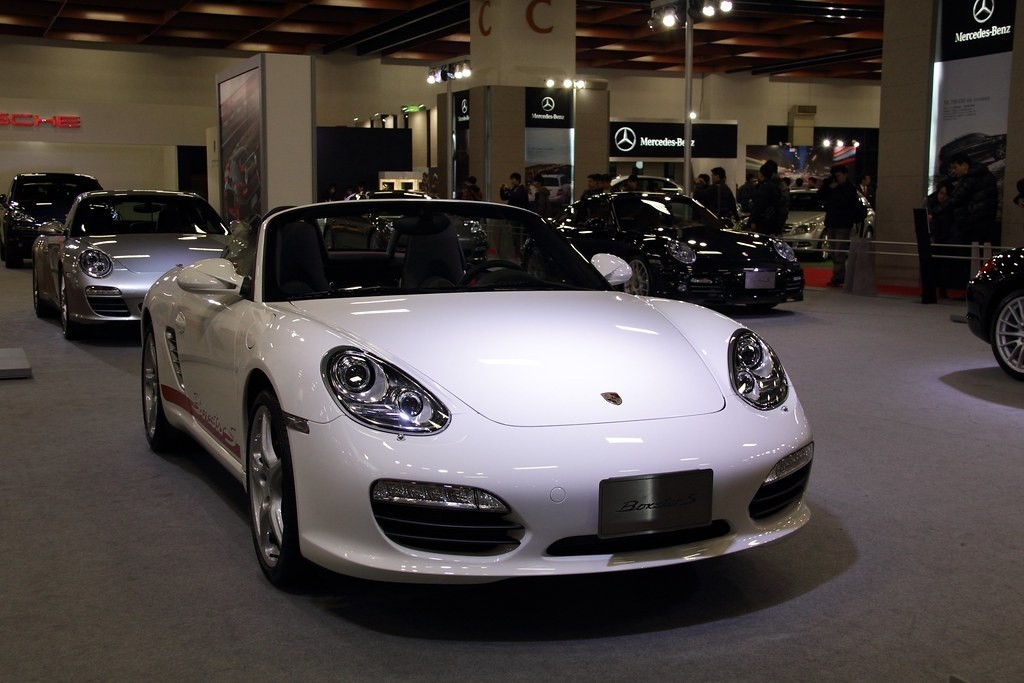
[647,0,734,224]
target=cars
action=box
[528,174,574,202]
[610,175,694,223]
[225,147,261,205]
[733,189,876,262]
[549,191,805,312]
[0,173,104,269]
[938,132,1007,175]
[323,190,489,263]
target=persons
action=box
[816,165,877,287]
[792,178,803,187]
[807,177,817,189]
[455,176,482,200]
[499,172,529,257]
[580,173,638,198]
[693,167,736,216]
[534,174,552,215]
[923,153,999,246]
[739,160,790,236]
[344,183,366,200]
[320,185,337,202]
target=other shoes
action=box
[827,278,844,287]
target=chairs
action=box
[256,205,326,297]
[87,208,114,233]
[155,209,193,233]
[653,208,678,226]
[402,215,464,290]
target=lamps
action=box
[447,62,472,80]
[662,0,733,30]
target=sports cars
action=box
[32,190,232,340]
[141,200,815,585]
